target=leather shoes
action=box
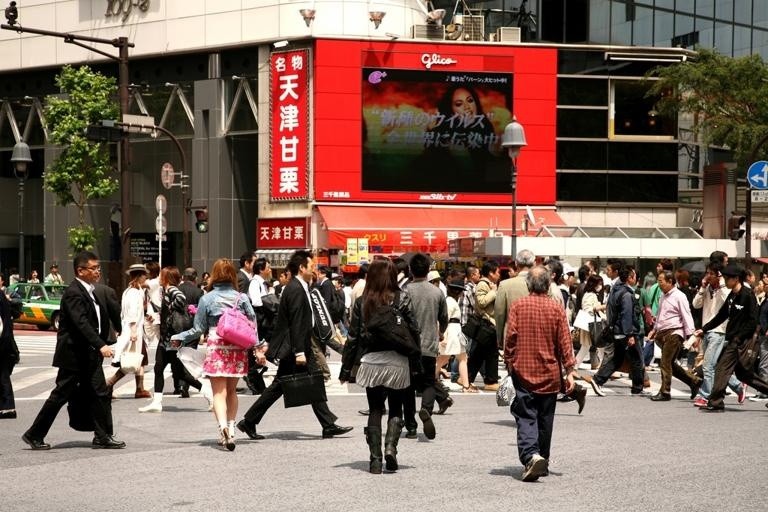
[322,425,353,439]
[0,410,17,420]
[93,434,126,448]
[236,420,266,440]
[690,378,704,399]
[651,392,672,401]
[575,388,587,415]
[359,407,385,415]
[583,360,591,363]
[180,386,189,398]
[699,405,725,413]
[22,430,50,450]
[438,397,454,414]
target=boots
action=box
[139,393,163,413]
[364,427,382,475]
[384,417,404,471]
[199,384,213,412]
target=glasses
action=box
[78,264,101,271]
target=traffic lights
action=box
[728,216,745,241]
[195,210,208,233]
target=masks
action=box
[596,285,602,292]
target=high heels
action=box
[217,421,234,446]
[439,371,451,379]
[219,422,235,450]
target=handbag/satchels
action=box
[164,310,192,335]
[600,322,615,343]
[216,292,261,350]
[462,314,497,349]
[176,346,206,380]
[737,336,760,370]
[68,384,113,431]
[4,289,23,320]
[366,290,421,358]
[570,328,580,342]
[643,306,655,326]
[120,341,144,374]
[655,329,675,348]
[566,296,576,323]
[573,308,602,332]
[0,334,20,364]
[281,365,328,408]
[588,307,610,347]
[496,374,516,406]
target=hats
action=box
[125,264,150,275]
[320,266,332,275]
[448,279,466,291]
[50,264,58,269]
[426,271,444,282]
[722,267,741,275]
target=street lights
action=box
[9,134,35,280]
[500,115,528,261]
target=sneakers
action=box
[694,398,709,406]
[749,395,768,402]
[419,408,435,439]
[434,378,449,391]
[457,378,474,390]
[462,385,478,393]
[632,391,652,396]
[591,379,605,397]
[522,452,546,481]
[135,390,150,398]
[406,429,416,439]
[484,384,501,391]
[582,375,594,384]
[738,381,747,403]
[644,380,650,387]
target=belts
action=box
[449,318,460,323]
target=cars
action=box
[5,283,71,330]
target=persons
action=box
[170,258,265,450]
[339,257,422,472]
[425,87,511,155]
[20,251,126,450]
[503,264,576,481]
[235,250,353,438]
[401,253,448,438]
[1,244,767,415]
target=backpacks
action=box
[326,280,345,323]
